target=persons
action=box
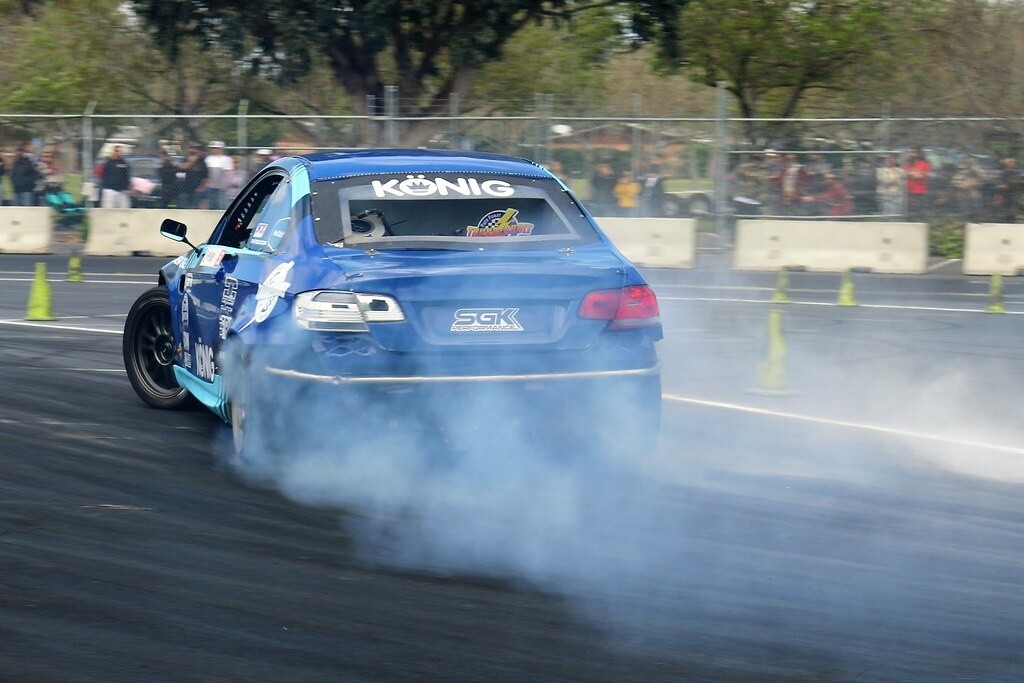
[158,140,274,209]
[725,131,1024,224]
[100,145,131,208]
[550,155,664,217]
[0,143,61,206]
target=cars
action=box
[123,148,665,471]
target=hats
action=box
[208,139,225,149]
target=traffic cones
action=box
[978,272,1008,316]
[768,269,792,304]
[742,308,803,397]
[21,263,61,321]
[64,257,84,282]
[835,272,860,307]
